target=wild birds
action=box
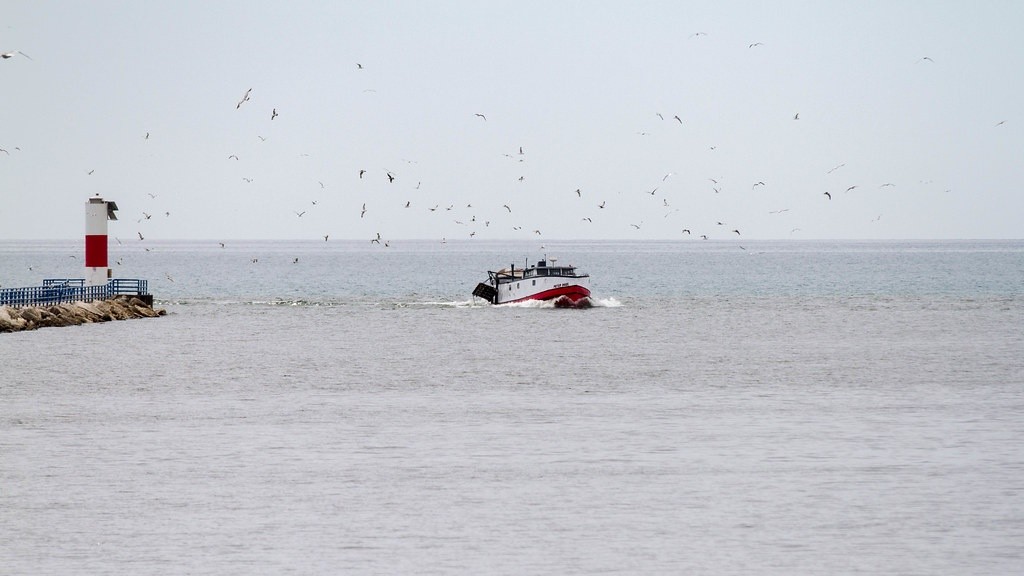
[878,183,896,188]
[916,57,935,64]
[995,119,1011,127]
[0,40,861,283]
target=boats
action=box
[486,256,591,306]
